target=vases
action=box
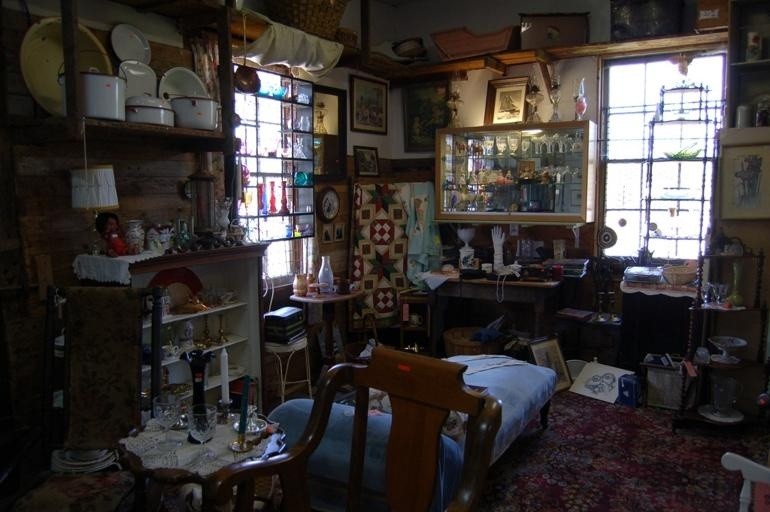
[726,258,750,309]
[175,348,218,445]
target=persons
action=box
[491,226,505,271]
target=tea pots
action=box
[707,371,745,419]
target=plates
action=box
[233,417,269,434]
[21,20,111,117]
[110,24,153,67]
[118,60,157,100]
[159,67,209,101]
[51,449,114,476]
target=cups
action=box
[160,345,179,361]
[480,263,492,274]
[699,281,732,305]
[553,265,566,280]
[472,257,479,268]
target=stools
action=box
[263,335,315,408]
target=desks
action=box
[290,285,366,383]
[427,275,563,351]
[552,308,622,350]
[115,403,292,512]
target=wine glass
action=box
[243,192,253,217]
[547,165,583,184]
[185,404,217,460]
[153,393,185,451]
[483,133,529,156]
[532,133,574,154]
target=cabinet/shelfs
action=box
[721,0,769,141]
[63,1,233,250]
[620,279,702,378]
[679,237,770,431]
[637,80,716,275]
[433,119,598,227]
[230,61,316,245]
[68,241,270,426]
[400,287,432,349]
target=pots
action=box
[122,92,176,127]
[171,97,221,132]
[55,72,126,123]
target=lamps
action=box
[66,160,121,258]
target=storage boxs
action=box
[608,0,698,44]
[623,265,663,285]
[641,351,696,412]
[262,304,306,348]
[518,10,591,51]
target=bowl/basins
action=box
[255,84,273,95]
[270,86,288,98]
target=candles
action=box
[220,346,232,404]
[238,371,252,434]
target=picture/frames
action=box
[348,73,390,136]
[487,75,533,125]
[321,223,334,244]
[401,76,452,153]
[527,333,573,396]
[716,140,770,224]
[353,145,382,178]
[334,222,346,242]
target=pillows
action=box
[368,386,467,441]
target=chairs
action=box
[1,279,168,509]
[200,344,505,512]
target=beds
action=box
[259,353,559,511]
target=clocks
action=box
[314,184,342,226]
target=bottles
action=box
[277,135,307,160]
[257,178,293,215]
[306,274,316,293]
[458,170,513,184]
[318,254,334,293]
[290,92,312,132]
